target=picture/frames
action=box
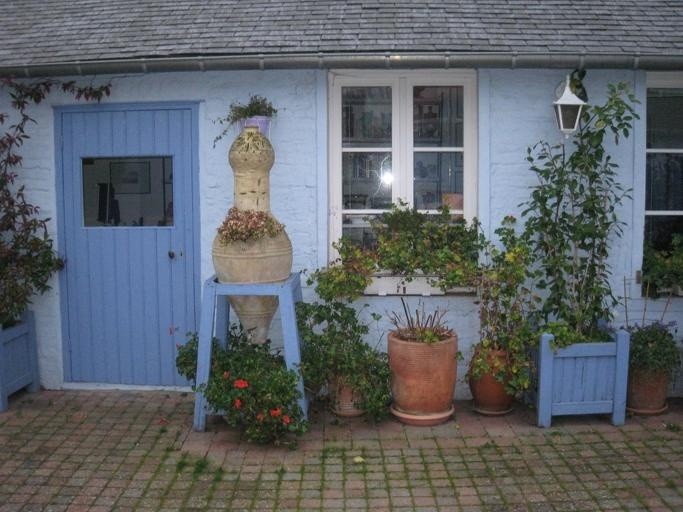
[109,161,151,195]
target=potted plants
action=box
[211,90,286,148]
[295,80,683,428]
[0,71,114,412]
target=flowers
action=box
[167,322,310,450]
[216,207,286,247]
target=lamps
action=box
[552,69,587,139]
